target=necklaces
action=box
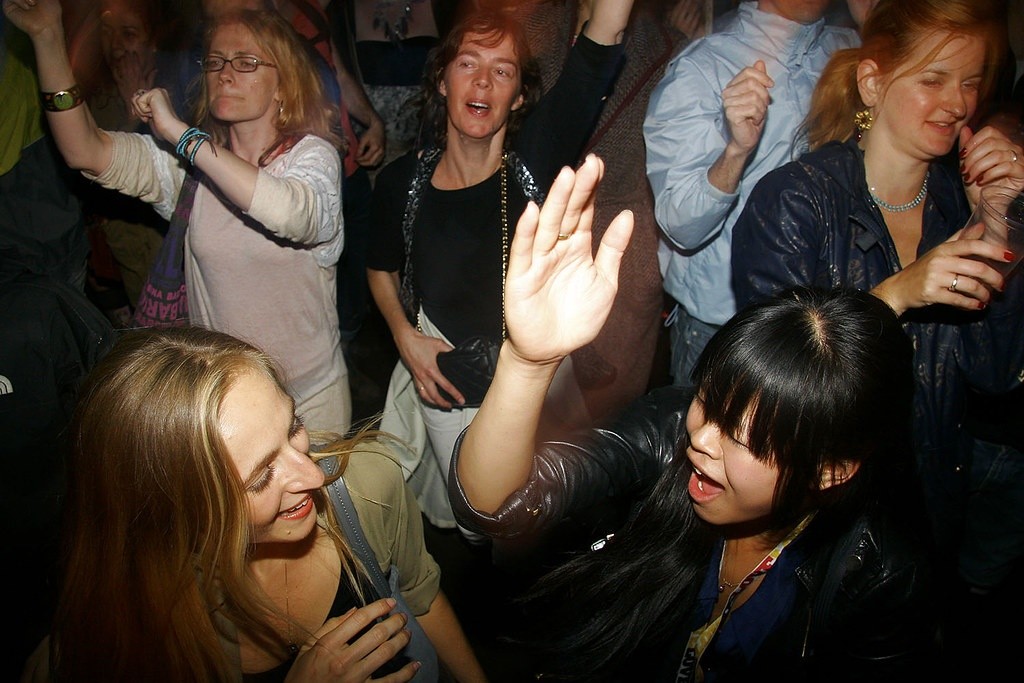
[283,557,299,654]
[870,171,929,211]
[718,556,740,592]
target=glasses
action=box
[197,55,277,73]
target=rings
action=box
[1008,149,1017,163]
[948,274,958,291]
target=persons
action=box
[18,322,488,683]
[732,0,1022,653]
[3,0,877,549]
[450,152,925,683]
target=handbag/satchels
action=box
[129,162,198,335]
[410,338,496,411]
